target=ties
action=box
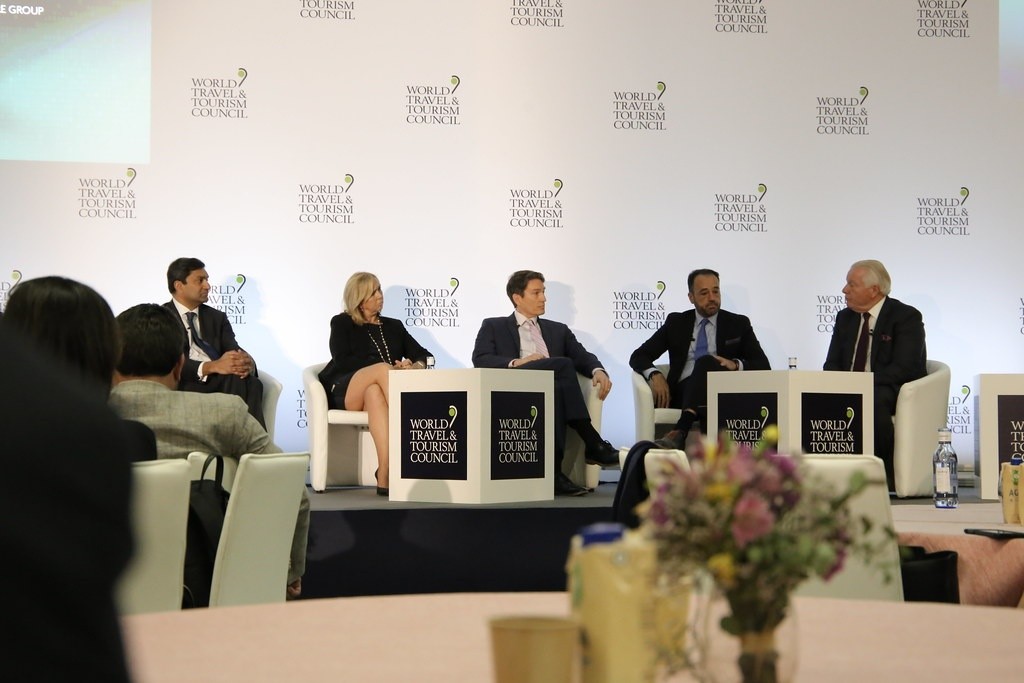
[527,321,549,359]
[185,312,220,362]
[694,319,710,360]
[853,311,872,371]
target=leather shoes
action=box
[585,440,620,469]
[554,472,588,496]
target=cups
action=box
[489,617,584,683]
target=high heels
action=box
[375,468,388,497]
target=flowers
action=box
[631,425,911,683]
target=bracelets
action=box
[649,371,661,379]
[734,360,739,370]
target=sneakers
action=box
[655,430,685,451]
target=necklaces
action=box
[368,318,392,365]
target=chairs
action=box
[257,365,283,445]
[631,364,682,445]
[893,360,950,498]
[112,452,311,614]
[561,369,603,493]
[302,362,379,493]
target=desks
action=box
[116,591,1024,683]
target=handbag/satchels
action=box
[182,452,231,609]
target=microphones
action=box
[870,329,873,332]
[187,327,191,329]
[517,324,520,328]
[688,335,695,341]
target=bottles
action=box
[426,356,434,369]
[788,357,797,370]
[933,428,959,508]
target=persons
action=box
[898,531,1023,607]
[106,303,310,601]
[472,271,620,496]
[318,272,435,496]
[629,269,772,450]
[4,276,216,601]
[823,260,928,458]
[161,257,267,431]
